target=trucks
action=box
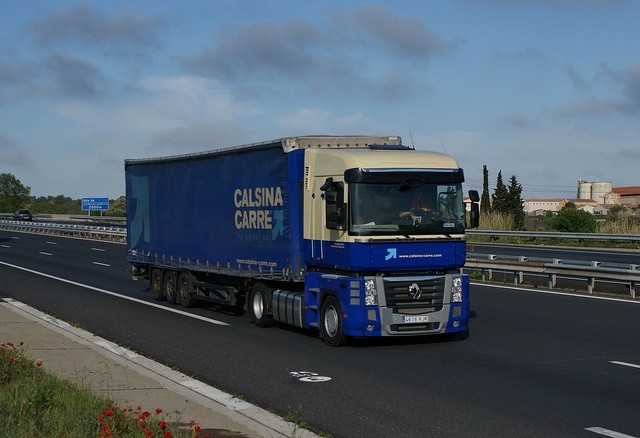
[124,134,479,348]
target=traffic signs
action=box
[81,197,109,210]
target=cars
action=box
[13,209,32,221]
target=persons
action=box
[399,187,431,217]
